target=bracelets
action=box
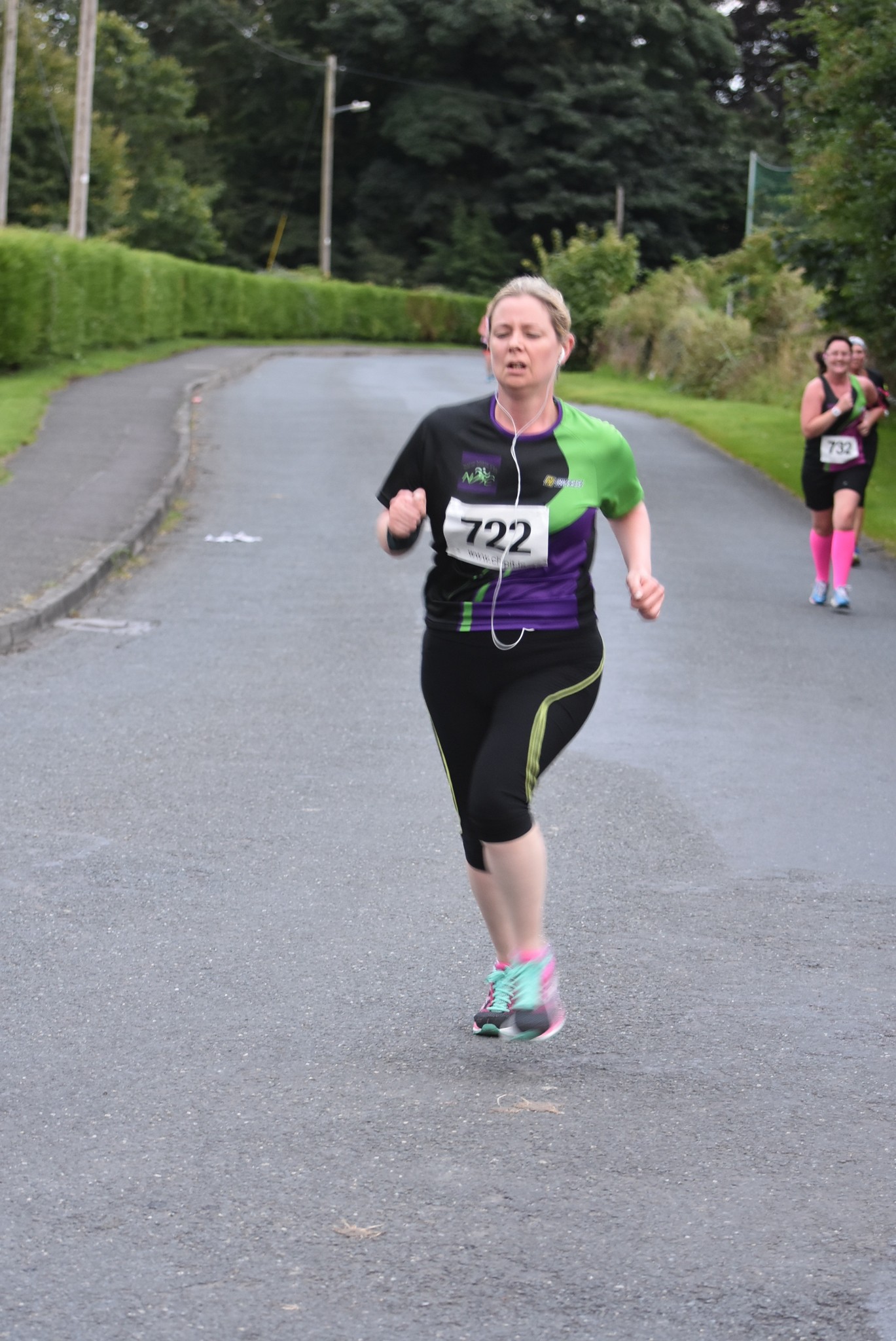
[832,406,841,418]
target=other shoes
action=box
[853,543,860,564]
[486,375,495,383]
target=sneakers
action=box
[831,584,849,609]
[472,959,518,1035]
[809,577,829,605]
[498,941,565,1042]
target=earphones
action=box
[558,348,565,364]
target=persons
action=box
[373,274,667,1044]
[799,335,890,613]
